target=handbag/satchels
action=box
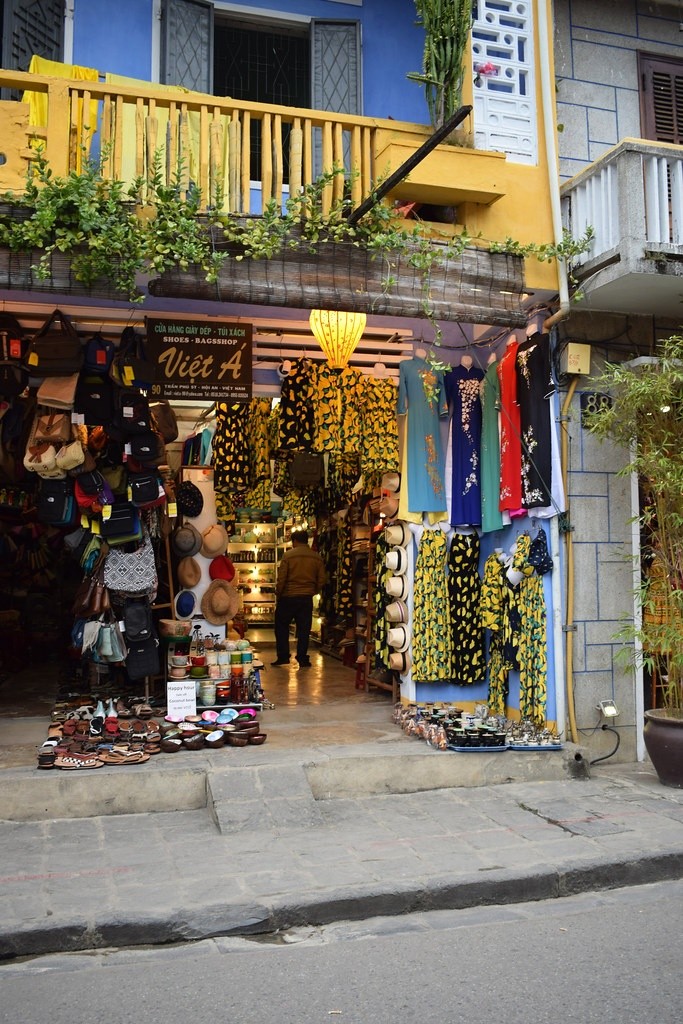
[0,309,179,676]
[287,451,323,488]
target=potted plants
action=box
[583,336,683,790]
[376,0,507,205]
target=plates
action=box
[190,675,207,678]
[169,674,190,680]
[171,663,189,668]
[190,665,207,667]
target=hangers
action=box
[525,518,542,536]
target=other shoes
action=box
[38,685,166,771]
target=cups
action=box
[220,665,231,679]
[208,666,220,678]
[231,664,242,677]
[218,651,230,665]
[231,651,241,664]
[243,664,252,678]
[207,652,218,666]
[241,652,252,664]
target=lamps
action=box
[600,700,620,717]
[309,309,367,375]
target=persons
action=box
[362,323,555,728]
[270,531,326,667]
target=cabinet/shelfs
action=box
[223,518,321,642]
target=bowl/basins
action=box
[191,667,206,675]
[171,668,186,677]
[162,710,267,752]
[191,657,204,665]
[200,680,229,706]
[173,656,186,666]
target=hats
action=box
[337,470,411,677]
[171,481,239,626]
